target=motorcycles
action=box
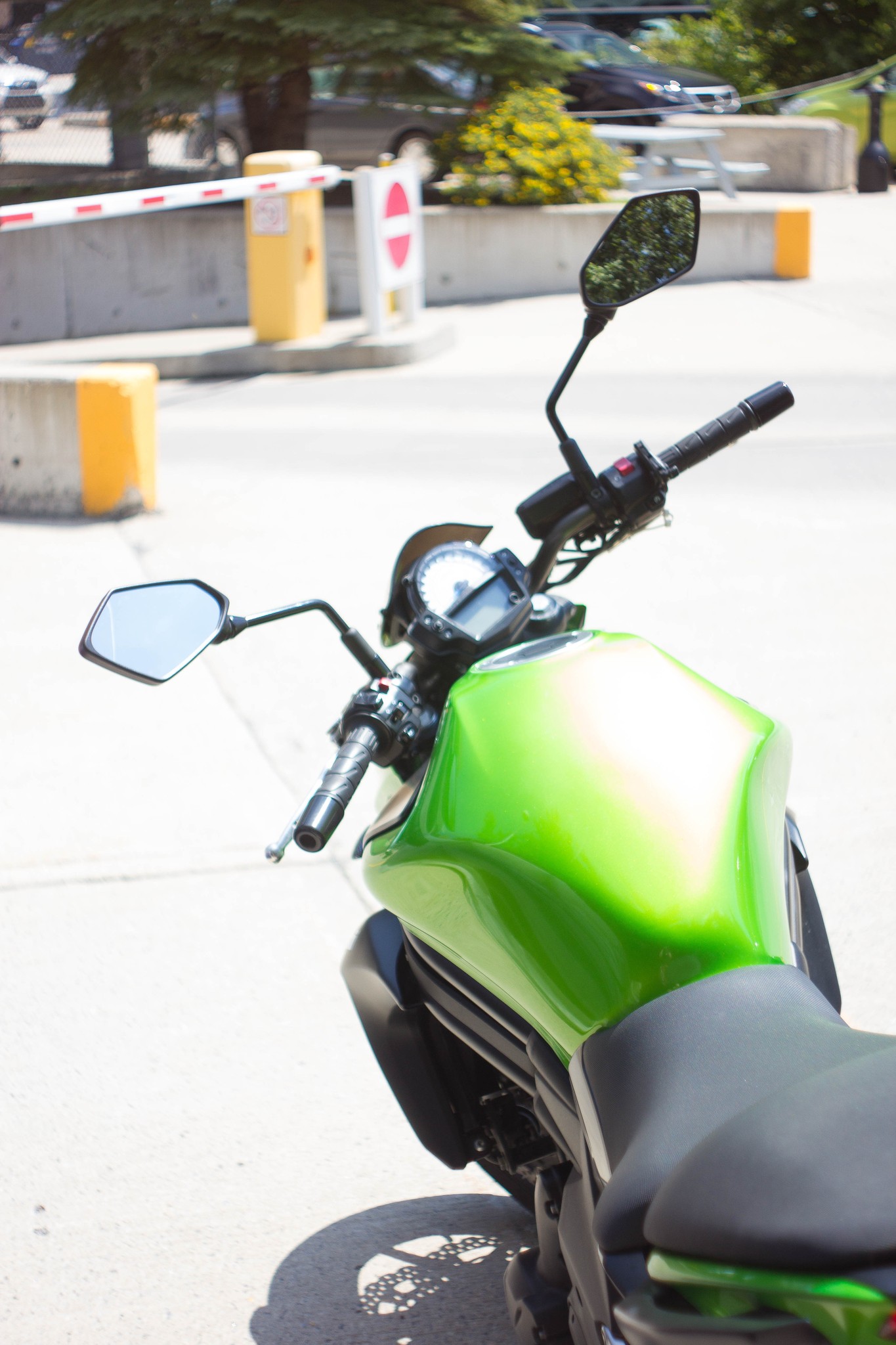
[74,187,896,1344]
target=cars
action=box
[518,19,745,155]
[0,44,76,132]
[183,56,490,187]
[780,54,896,168]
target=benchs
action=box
[614,154,772,191]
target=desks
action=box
[592,123,738,197]
